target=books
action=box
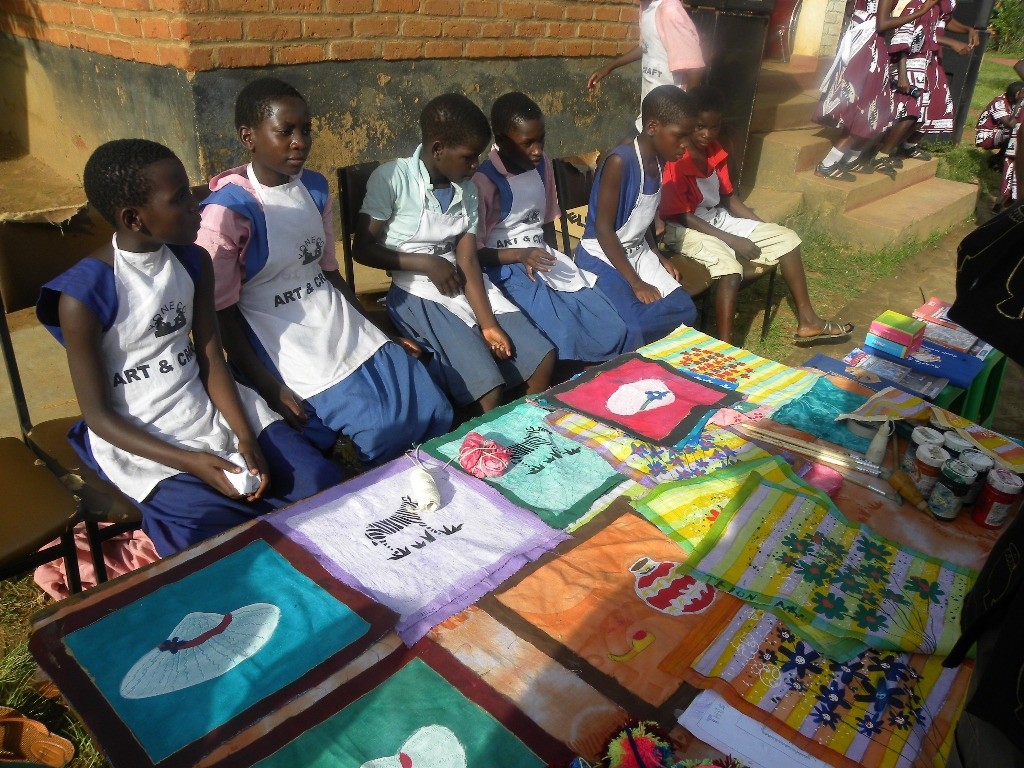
[913,298,979,353]
[864,310,925,359]
[841,347,951,400]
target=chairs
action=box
[0,150,780,590]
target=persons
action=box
[657,86,855,345]
[586,0,708,136]
[975,81,1023,150]
[352,92,557,417]
[892,0,979,161]
[871,0,939,176]
[571,84,700,344]
[992,58,1024,212]
[194,75,456,463]
[810,0,937,181]
[470,93,627,375]
[35,138,348,557]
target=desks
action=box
[32,321,1024,768]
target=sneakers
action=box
[869,156,897,177]
[814,161,858,182]
[872,145,904,168]
[896,143,932,161]
[846,159,874,174]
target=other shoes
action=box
[991,204,1004,214]
[989,154,1003,173]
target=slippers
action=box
[793,319,856,342]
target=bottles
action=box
[903,420,1024,536]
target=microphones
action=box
[894,84,921,99]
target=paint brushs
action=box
[738,422,907,506]
